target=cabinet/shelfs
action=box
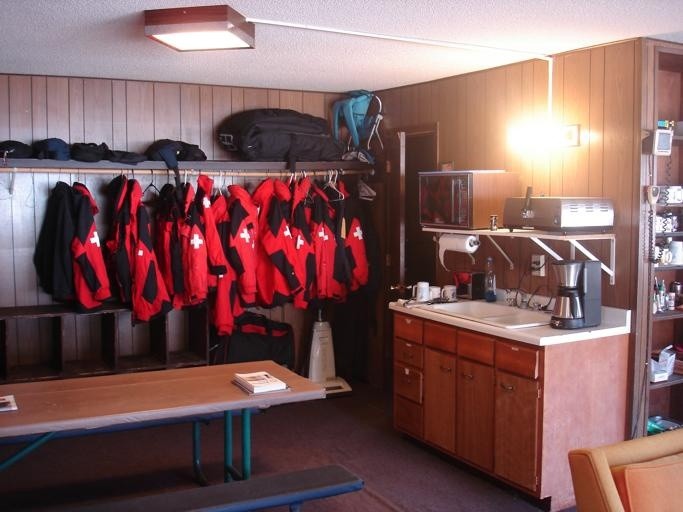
[393,314,423,442]
[389,275,632,512]
[425,320,494,476]
[1,169,378,379]
[496,336,539,500]
[648,135,683,392]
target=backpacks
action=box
[217,108,348,173]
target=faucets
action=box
[506,287,529,310]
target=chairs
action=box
[567,426,682,511]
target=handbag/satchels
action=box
[229,310,294,369]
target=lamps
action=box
[143,0,256,52]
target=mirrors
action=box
[398,120,440,297]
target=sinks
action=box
[417,300,553,329]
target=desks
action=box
[1,355,327,478]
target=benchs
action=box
[96,463,365,512]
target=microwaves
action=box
[418,169,523,232]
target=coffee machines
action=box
[548,258,603,329]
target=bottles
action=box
[669,240,682,266]
[489,215,498,231]
[652,281,665,315]
[664,237,673,249]
[668,292,676,310]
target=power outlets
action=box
[530,253,546,277]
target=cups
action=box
[655,216,679,233]
[429,285,441,300]
[666,185,682,204]
[411,282,430,302]
[654,246,671,266]
[441,285,458,300]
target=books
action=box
[0,393,19,412]
[228,369,293,396]
